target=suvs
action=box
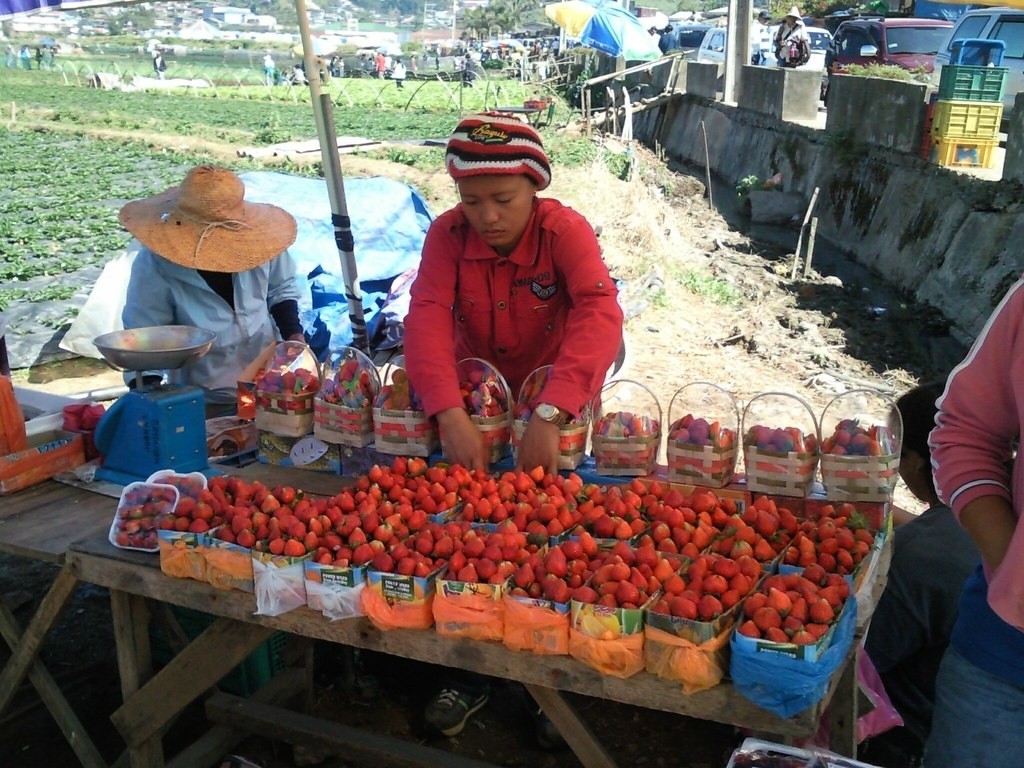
[825,15,953,77]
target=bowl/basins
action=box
[108,469,211,553]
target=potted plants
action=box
[826,61,930,152]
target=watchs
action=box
[534,402,566,428]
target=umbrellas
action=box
[544,0,663,62]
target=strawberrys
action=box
[115,476,202,548]
[457,369,549,418]
[596,410,899,456]
[255,357,418,412]
[159,453,738,609]
[652,493,873,643]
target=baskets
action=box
[516,366,589,472]
[372,355,440,456]
[818,384,905,502]
[251,342,321,436]
[312,349,382,447]
[744,392,819,498]
[588,379,663,475]
[666,382,740,488]
[443,358,514,465]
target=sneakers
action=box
[522,685,571,750]
[423,676,487,737]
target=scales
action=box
[92,324,221,486]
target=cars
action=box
[671,24,714,61]
[695,27,761,93]
[762,26,832,99]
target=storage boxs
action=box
[237,340,284,419]
[524,96,552,109]
[157,501,884,663]
[921,65,1011,169]
[258,431,894,532]
[169,603,290,694]
[0,386,98,496]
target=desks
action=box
[0,478,108,768]
[69,472,893,768]
[496,107,550,129]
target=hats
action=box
[760,10,773,19]
[444,111,552,192]
[785,6,803,20]
[118,165,296,273]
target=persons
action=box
[774,6,808,68]
[5,41,59,71]
[922,274,1024,768]
[403,112,624,476]
[864,382,980,768]
[750,10,771,65]
[153,51,168,79]
[119,164,308,420]
[220,12,679,90]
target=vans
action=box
[932,8,1024,125]
